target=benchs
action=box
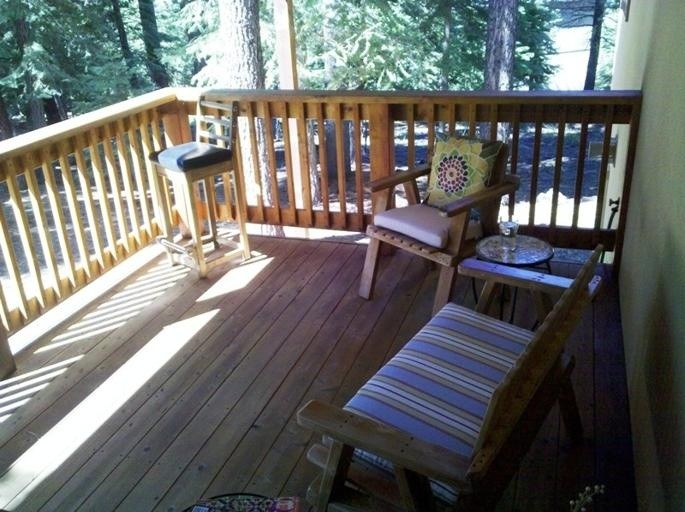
[296,243,607,512]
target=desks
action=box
[471,234,555,331]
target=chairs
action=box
[356,141,521,318]
[147,95,252,278]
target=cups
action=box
[499,215,521,253]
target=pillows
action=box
[422,132,504,219]
